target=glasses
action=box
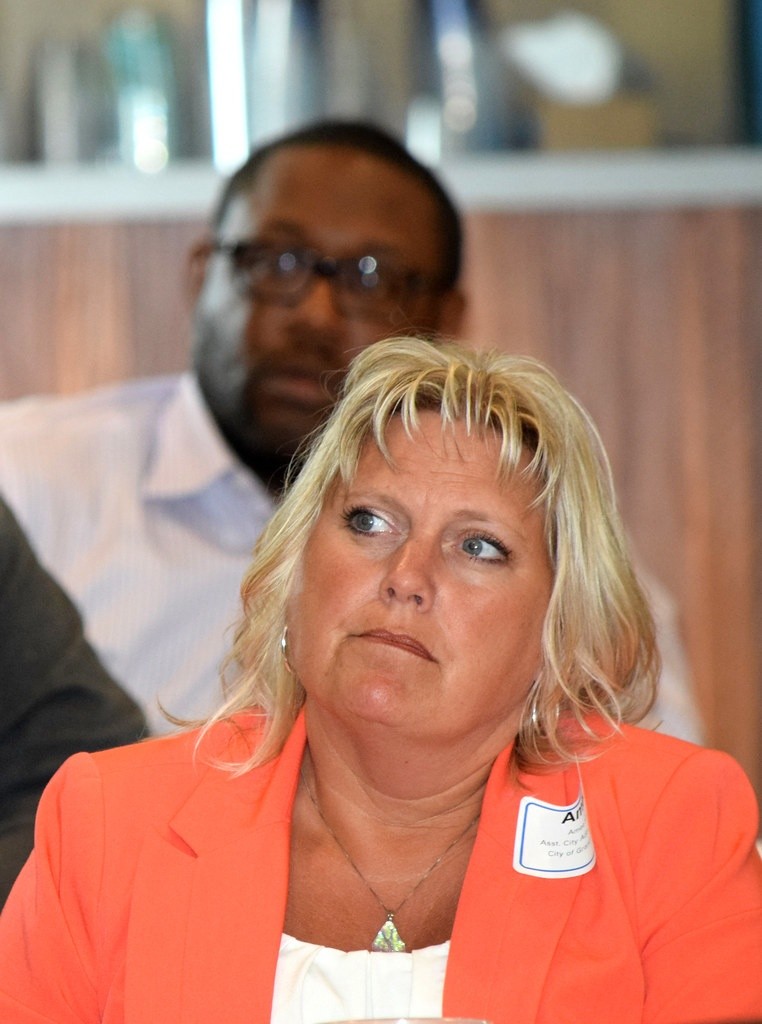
[203,233,445,319]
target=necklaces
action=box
[298,769,481,953]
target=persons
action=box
[0,112,708,749]
[0,493,151,912]
[0,323,761,1024]
